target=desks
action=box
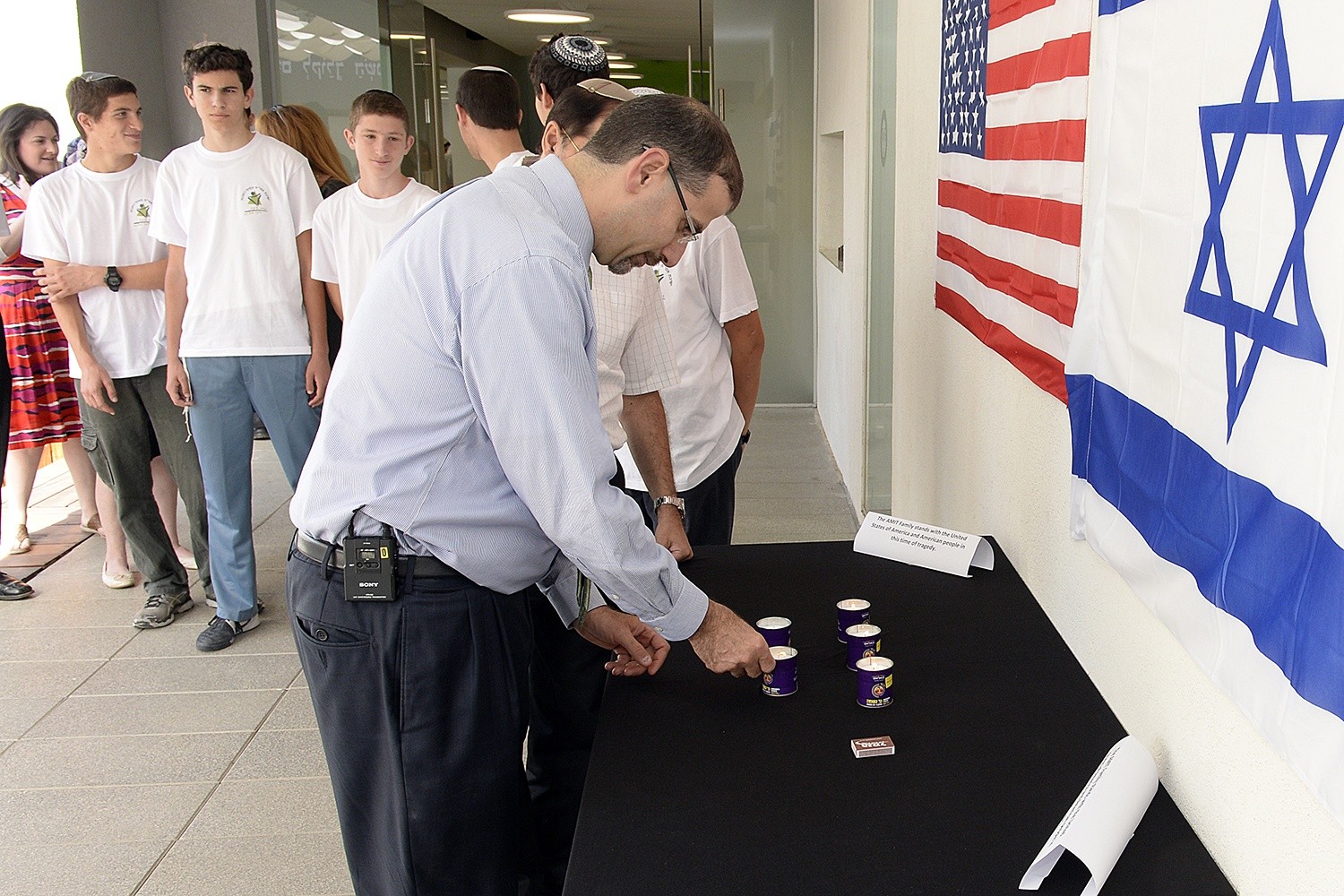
[559,536,1236,895]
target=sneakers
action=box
[206,595,266,613]
[196,613,260,652]
[133,590,194,628]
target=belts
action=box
[295,530,461,577]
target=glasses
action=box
[643,144,699,244]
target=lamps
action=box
[537,35,614,44]
[504,8,595,24]
[609,73,645,79]
[609,61,637,69]
[606,52,627,59]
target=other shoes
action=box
[174,545,199,569]
[102,558,134,589]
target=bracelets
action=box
[740,432,750,444]
[652,496,685,520]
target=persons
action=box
[257,103,356,369]
[613,214,766,547]
[0,103,105,554]
[387,78,694,895]
[0,311,34,600]
[62,137,198,588]
[309,88,441,320]
[284,89,776,895]
[243,107,269,440]
[20,72,265,628]
[146,40,329,652]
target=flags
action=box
[934,0,1344,824]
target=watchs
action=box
[103,266,122,292]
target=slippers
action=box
[81,513,106,538]
[4,524,30,554]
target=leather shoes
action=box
[0,570,34,600]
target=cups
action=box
[756,617,793,649]
[836,599,871,644]
[845,624,882,671]
[761,645,799,696]
[855,657,893,708]
